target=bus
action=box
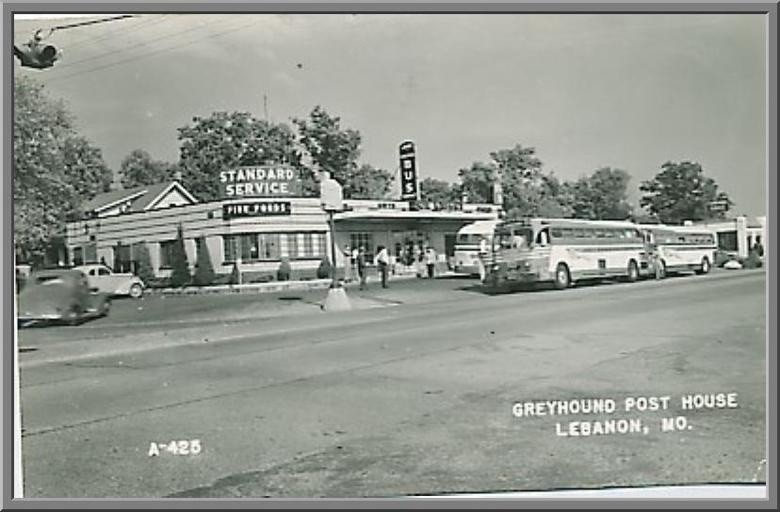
[453,219,718,290]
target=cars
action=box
[71,264,145,299]
[18,268,111,326]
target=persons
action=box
[348,239,437,290]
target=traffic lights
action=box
[13,28,63,69]
